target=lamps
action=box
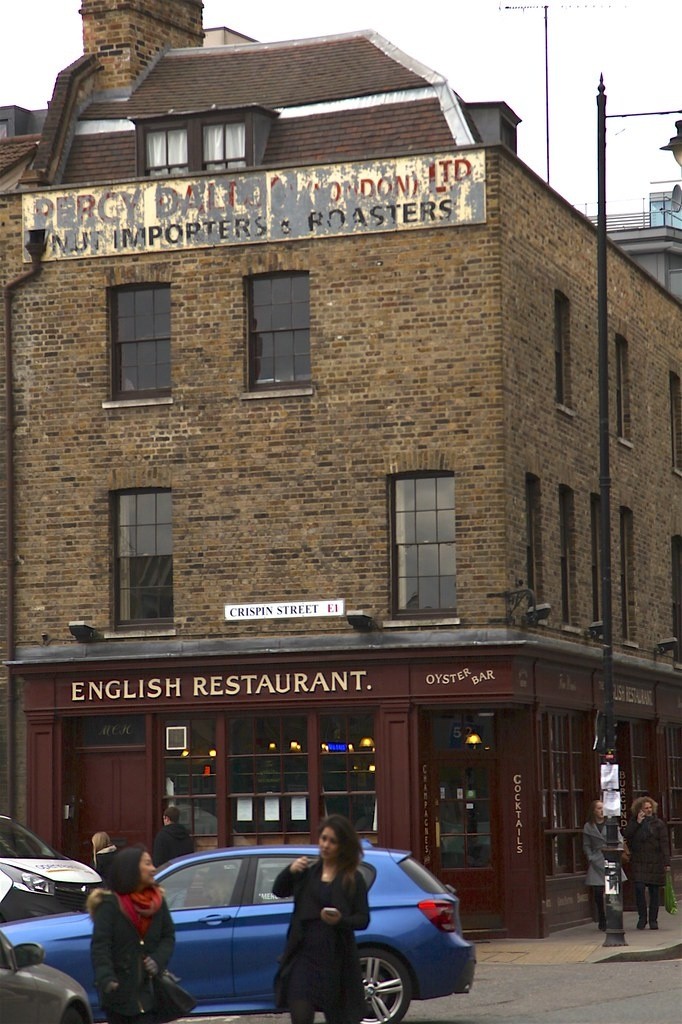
[588,620,603,634]
[67,620,96,643]
[526,603,553,626]
[658,637,677,653]
[346,610,375,632]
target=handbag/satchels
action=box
[664,871,678,915]
[139,970,197,1022]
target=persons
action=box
[92,832,118,890]
[624,796,672,930]
[86,847,197,1024]
[271,815,371,1024]
[152,807,194,868]
[583,800,628,931]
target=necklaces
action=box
[322,865,331,877]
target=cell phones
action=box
[325,908,337,917]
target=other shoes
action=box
[637,918,647,929]
[649,920,658,929]
[598,920,606,931]
